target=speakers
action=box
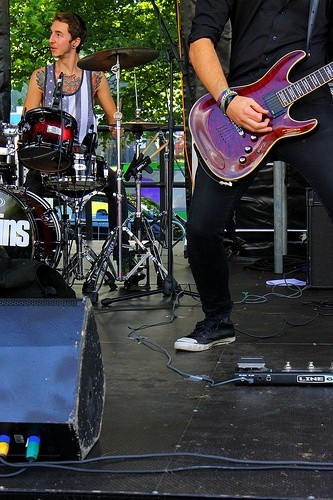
[0,296,106,461]
[306,203,333,289]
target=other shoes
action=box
[118,262,146,281]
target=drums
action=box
[0,184,65,269]
[41,152,106,190]
[17,107,77,172]
[0,162,17,184]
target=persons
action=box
[174,0,333,352]
[24,13,146,281]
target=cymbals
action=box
[77,47,160,71]
[121,118,160,130]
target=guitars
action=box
[189,49,333,180]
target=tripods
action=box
[55,55,170,294]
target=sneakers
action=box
[173,316,236,351]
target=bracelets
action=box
[217,88,237,112]
